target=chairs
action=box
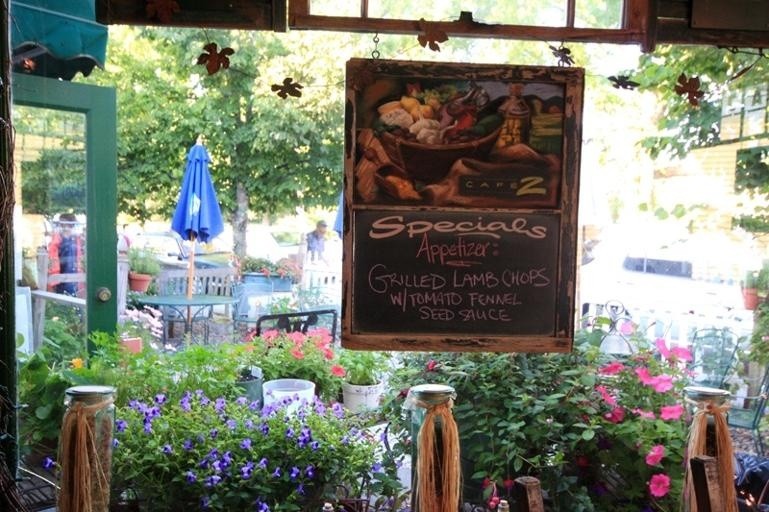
[678,336,769,457]
[256,310,337,345]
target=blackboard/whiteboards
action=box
[341,205,576,353]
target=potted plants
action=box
[126,245,161,293]
[742,259,769,310]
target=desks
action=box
[137,294,240,353]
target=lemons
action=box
[400,95,421,113]
[412,104,434,122]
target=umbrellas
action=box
[169,133,225,338]
[331,191,343,238]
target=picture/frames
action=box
[340,56,584,354]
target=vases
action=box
[241,271,294,292]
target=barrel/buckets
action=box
[341,378,384,414]
[261,379,316,411]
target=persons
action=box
[120,223,131,254]
[306,219,329,261]
[46,212,85,301]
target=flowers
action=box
[10,318,694,512]
[240,253,303,284]
